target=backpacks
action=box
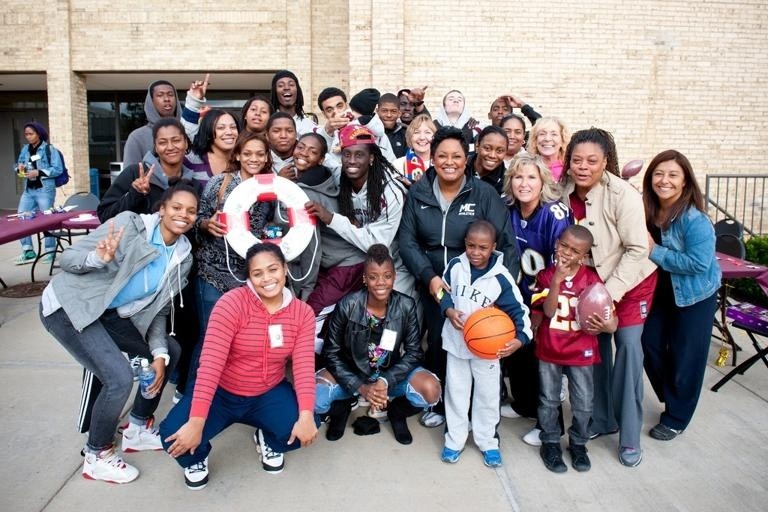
[46,145,68,187]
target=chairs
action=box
[50,192,100,275]
[711,219,768,392]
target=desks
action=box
[0,209,94,294]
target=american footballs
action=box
[622,160,643,177]
[576,282,612,336]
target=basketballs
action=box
[463,308,516,360]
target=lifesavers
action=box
[219,174,315,264]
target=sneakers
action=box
[81,451,141,484]
[621,445,643,466]
[13,251,57,265]
[482,451,502,468]
[420,412,444,427]
[121,424,165,452]
[184,458,211,490]
[252,428,286,474]
[569,443,591,471]
[539,443,566,472]
[442,443,466,464]
[500,403,542,446]
[648,422,686,442]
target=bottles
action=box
[138,359,157,400]
[26,162,37,181]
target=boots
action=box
[319,401,351,441]
[387,396,423,445]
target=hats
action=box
[337,124,377,147]
[24,121,48,140]
[349,89,380,115]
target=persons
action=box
[38,67,727,492]
[11,120,65,265]
